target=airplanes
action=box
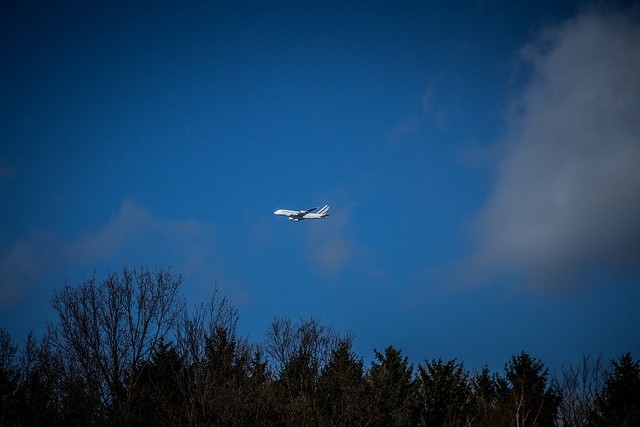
[274,204,331,222]
